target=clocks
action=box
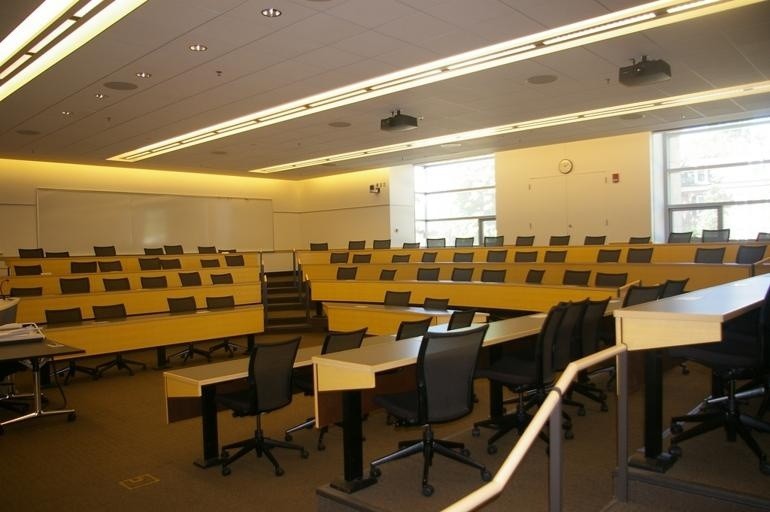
[558,159,573,174]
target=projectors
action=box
[618,59,673,87]
[381,114,419,132]
